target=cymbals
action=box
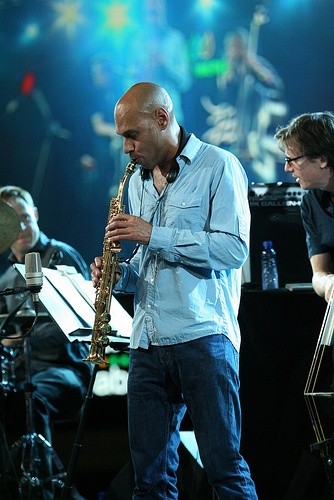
[0,308,48,318]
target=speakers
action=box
[242,181,313,286]
[239,288,334,500]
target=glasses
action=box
[285,155,304,167]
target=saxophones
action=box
[84,162,137,367]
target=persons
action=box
[178,24,289,183]
[90,82,259,500]
[278,111,334,309]
[0,186,92,500]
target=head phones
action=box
[139,124,187,183]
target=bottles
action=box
[261,241,279,291]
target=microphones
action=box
[24,252,43,314]
